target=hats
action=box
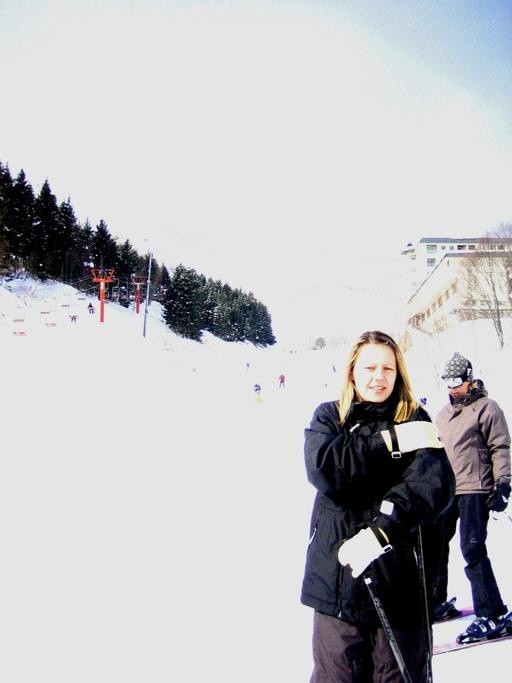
[440,351,473,382]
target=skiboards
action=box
[428,598,512,657]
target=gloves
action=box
[338,525,394,579]
[487,483,511,511]
[381,421,444,459]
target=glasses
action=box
[444,360,472,388]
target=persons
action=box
[88,303,93,313]
[434,350,512,639]
[279,374,285,388]
[300,331,456,683]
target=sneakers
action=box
[466,611,512,638]
[434,602,462,621]
